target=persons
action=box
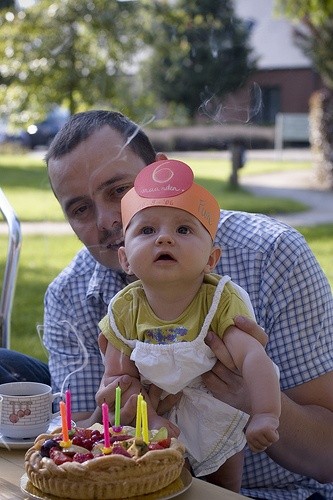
[42,110,333,500]
[95,160,282,494]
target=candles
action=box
[141,400,148,445]
[59,397,68,441]
[65,383,71,430]
[102,398,110,447]
[115,382,120,425]
[136,393,143,438]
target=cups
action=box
[0,382,65,439]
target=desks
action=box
[0,446,253,500]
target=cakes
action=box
[24,425,185,500]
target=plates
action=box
[0,415,76,448]
[20,467,190,499]
